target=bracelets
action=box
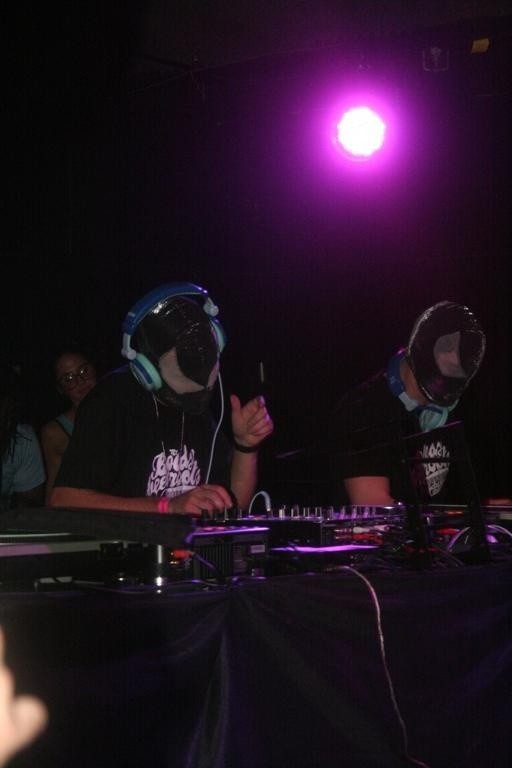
[235,443,259,454]
[156,496,169,515]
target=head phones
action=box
[378,350,459,433]
[122,282,227,393]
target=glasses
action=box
[55,362,98,392]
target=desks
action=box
[1,558,511,768]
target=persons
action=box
[308,297,488,519]
[43,279,277,525]
[0,365,49,513]
[0,609,52,768]
[36,334,111,497]
